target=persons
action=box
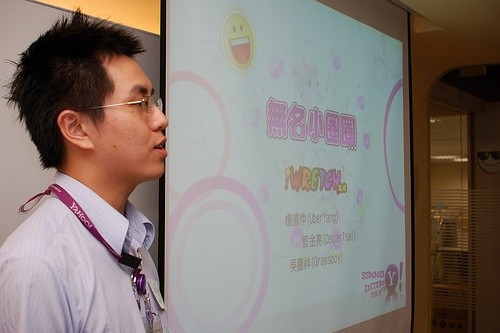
[0,5,168,333]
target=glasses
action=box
[74,96,163,113]
